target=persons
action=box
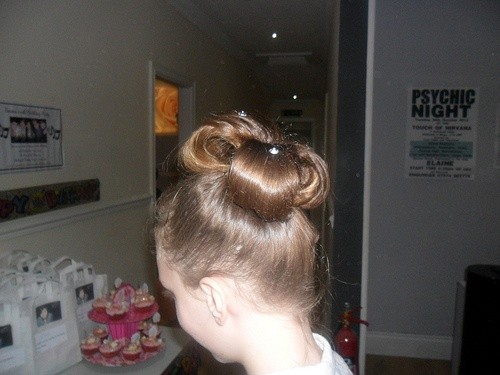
[37,307,52,327]
[148,111,354,375]
[77,289,88,304]
[10,120,46,139]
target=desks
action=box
[59,324,203,375]
